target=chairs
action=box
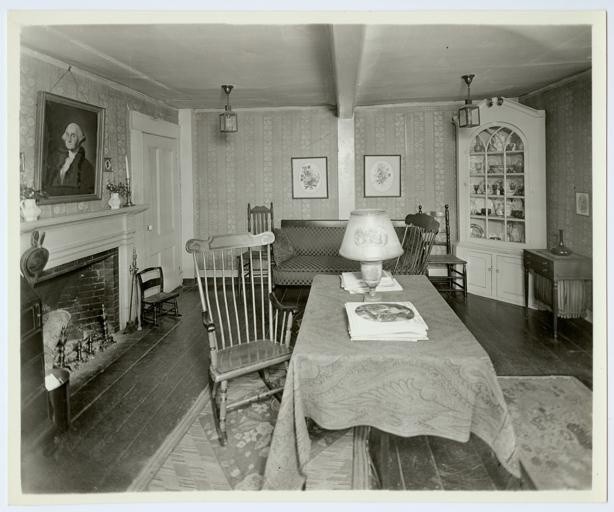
[185,231,298,446]
[419,203,468,303]
[137,267,182,328]
[236,202,274,303]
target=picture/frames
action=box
[291,156,329,200]
[33,89,106,207]
[363,154,402,198]
[575,191,591,218]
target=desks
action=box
[286,274,511,443]
[523,248,592,340]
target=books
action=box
[344,302,428,341]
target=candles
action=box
[125,154,129,178]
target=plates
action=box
[469,223,484,239]
[478,180,491,194]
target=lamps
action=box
[339,209,405,304]
[219,85,238,133]
[458,74,480,128]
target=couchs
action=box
[271,225,426,313]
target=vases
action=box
[20,199,41,222]
[107,192,121,210]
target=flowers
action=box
[105,181,127,195]
[21,186,50,200]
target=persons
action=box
[44,122,95,195]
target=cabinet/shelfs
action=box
[452,97,547,310]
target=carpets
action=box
[498,376,592,490]
[127,359,380,489]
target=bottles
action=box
[550,229,572,255]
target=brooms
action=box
[123,249,137,335]
[134,259,139,331]
